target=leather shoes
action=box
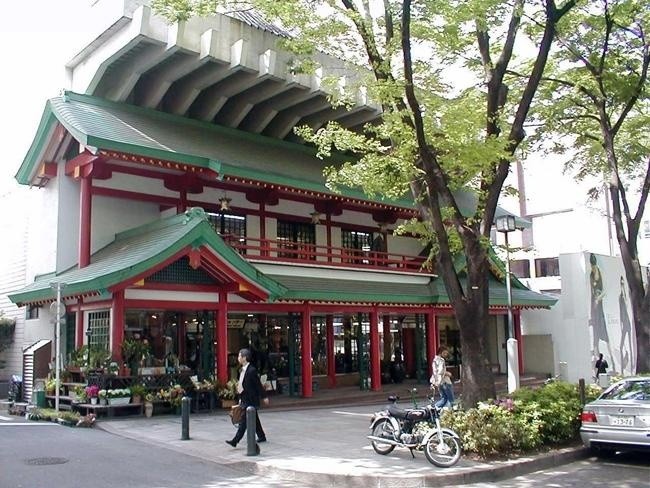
[226,439,238,447]
[257,438,267,442]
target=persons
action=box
[225,348,266,447]
[595,353,608,378]
[429,346,455,407]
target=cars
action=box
[579,375,650,459]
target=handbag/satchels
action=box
[430,374,444,387]
[230,405,243,424]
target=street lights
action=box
[493,214,517,338]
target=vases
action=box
[90,397,131,407]
[222,399,238,409]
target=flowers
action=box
[157,384,185,408]
[86,384,131,398]
[218,379,238,400]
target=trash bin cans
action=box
[381,359,407,385]
[33,391,45,407]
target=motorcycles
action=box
[366,375,461,468]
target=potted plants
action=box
[46,379,56,395]
[65,339,151,377]
[131,384,157,418]
[24,406,81,426]
